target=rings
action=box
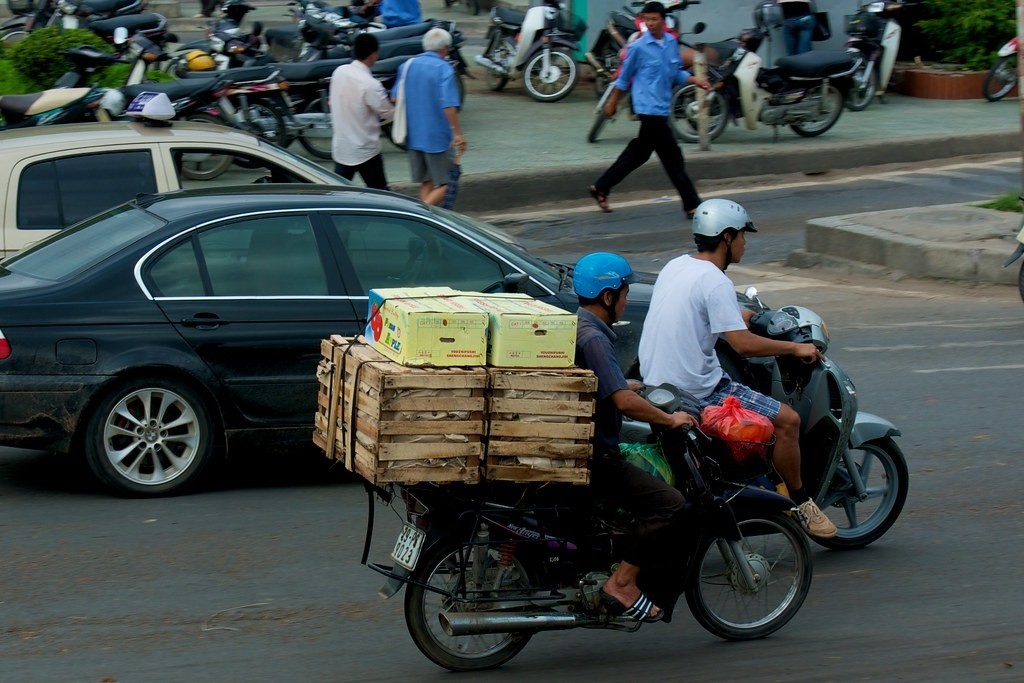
[817,349,819,355]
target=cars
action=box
[1,184,766,497]
[0,91,526,304]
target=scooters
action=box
[585,1,904,151]
[694,287,910,553]
[981,36,1021,101]
[1,0,471,159]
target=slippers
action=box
[599,586,666,622]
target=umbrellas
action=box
[435,137,463,209]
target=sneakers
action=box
[791,496,837,537]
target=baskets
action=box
[843,13,880,37]
[704,434,779,481]
[8,0,33,13]
[700,42,736,67]
[558,11,590,42]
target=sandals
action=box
[687,208,696,219]
[589,185,613,213]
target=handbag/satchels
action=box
[619,442,671,484]
[391,57,414,143]
[700,396,773,444]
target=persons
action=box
[362,0,423,30]
[637,197,841,537]
[776,0,815,56]
[328,32,395,190]
[587,1,712,220]
[570,252,701,623]
[390,29,467,207]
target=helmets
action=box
[691,198,758,236]
[572,252,631,298]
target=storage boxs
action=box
[315,288,591,489]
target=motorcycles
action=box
[360,380,819,676]
[473,0,591,104]
